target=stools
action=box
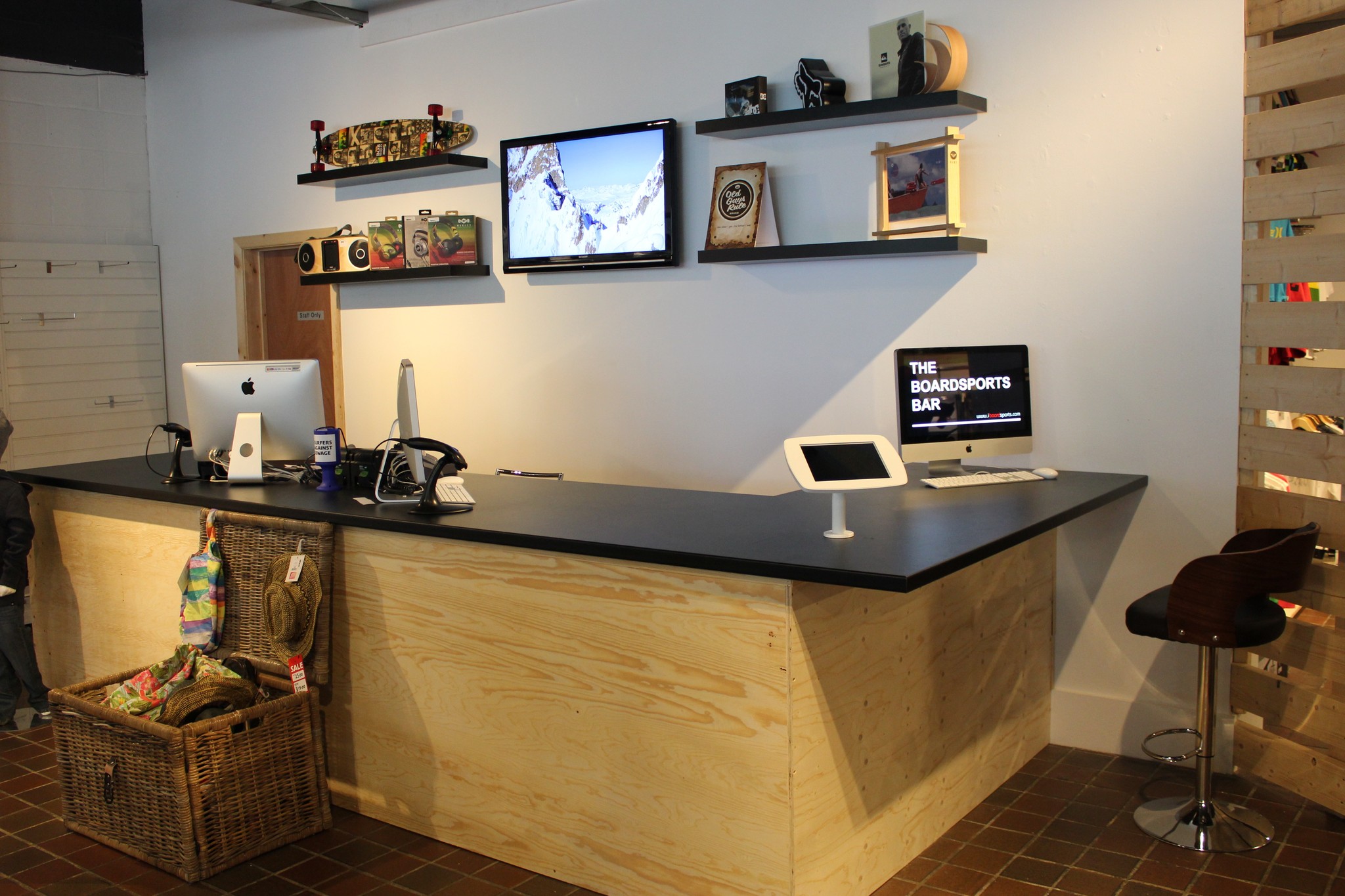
[1124,522,1321,852]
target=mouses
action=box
[1033,467,1058,479]
[436,476,464,484]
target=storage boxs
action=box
[368,216,406,271]
[48,507,350,880]
[402,210,434,268]
[427,210,479,269]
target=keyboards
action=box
[920,471,1044,489]
[435,484,476,504]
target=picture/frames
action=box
[870,126,967,240]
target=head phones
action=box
[414,230,429,256]
[373,223,403,260]
[433,218,463,258]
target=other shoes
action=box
[39,710,52,720]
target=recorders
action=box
[297,235,371,274]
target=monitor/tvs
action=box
[182,359,327,484]
[375,359,426,502]
[783,435,908,493]
[499,119,678,274]
[894,345,1033,478]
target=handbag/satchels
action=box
[177,509,225,652]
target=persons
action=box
[896,17,924,97]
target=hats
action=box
[153,673,257,728]
[261,552,322,666]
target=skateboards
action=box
[310,104,472,173]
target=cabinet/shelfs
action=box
[297,106,491,286]
[694,89,988,264]
